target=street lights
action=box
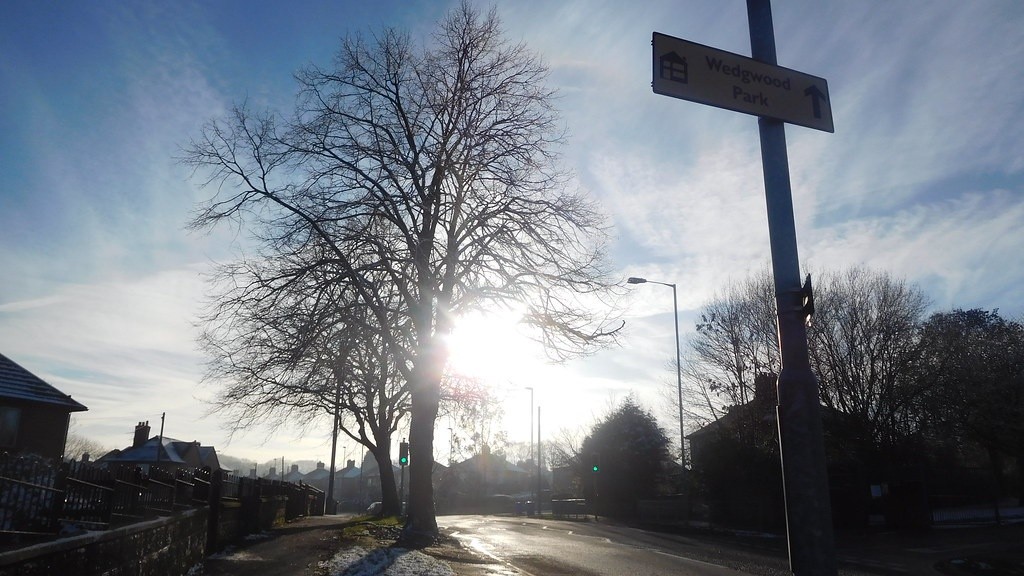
[628,277,686,477]
[523,386,534,514]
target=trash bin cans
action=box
[525,500,535,516]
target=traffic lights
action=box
[399,442,408,467]
